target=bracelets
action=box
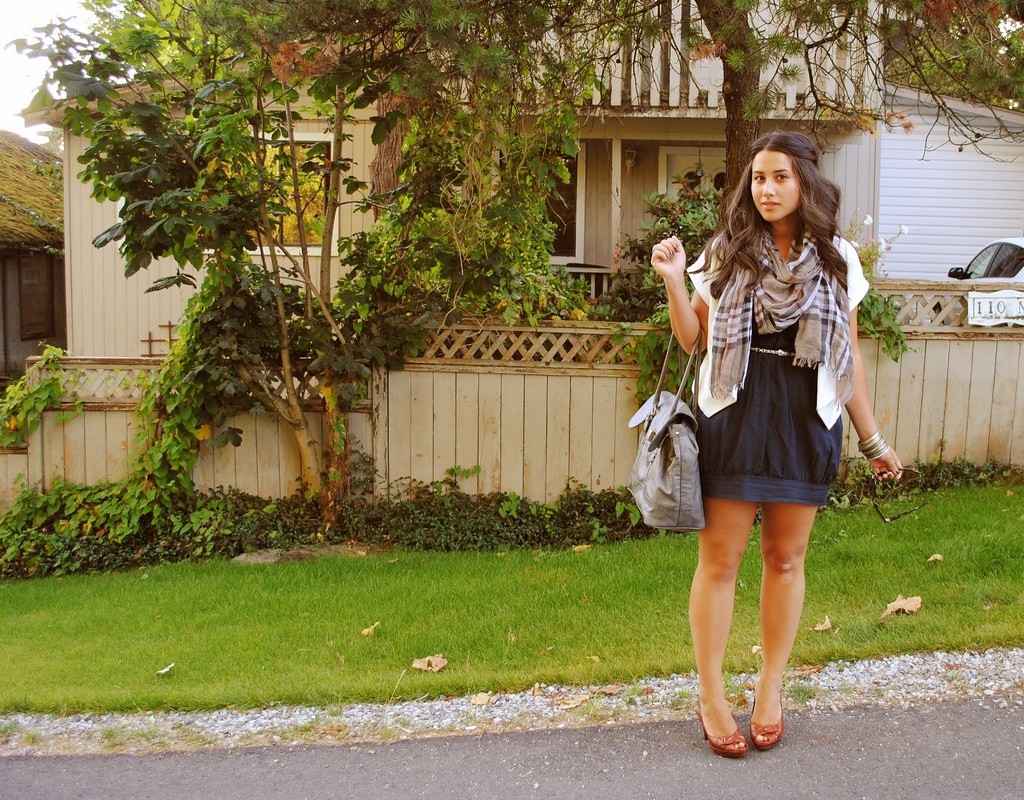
[857,431,890,462]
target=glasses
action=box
[867,469,931,523]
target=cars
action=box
[928,235,1024,330]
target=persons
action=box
[649,130,905,760]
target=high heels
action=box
[695,702,748,758]
[747,692,785,749]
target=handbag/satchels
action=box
[625,330,706,530]
